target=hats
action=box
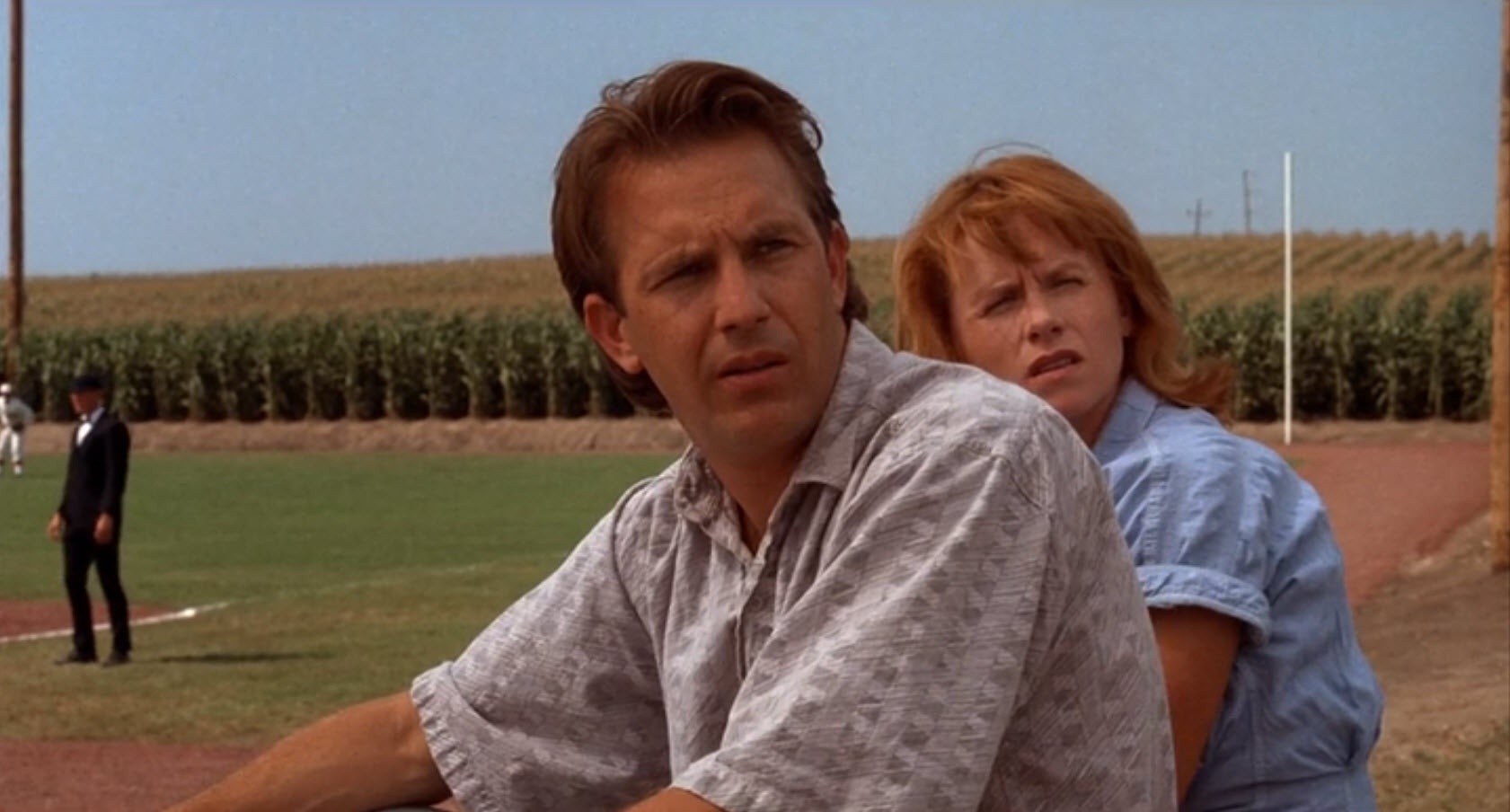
[57,373,102,392]
[0,383,12,394]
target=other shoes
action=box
[14,464,22,475]
[102,649,131,667]
[56,650,97,664]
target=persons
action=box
[0,383,37,478]
[157,55,1384,812]
[47,373,133,668]
